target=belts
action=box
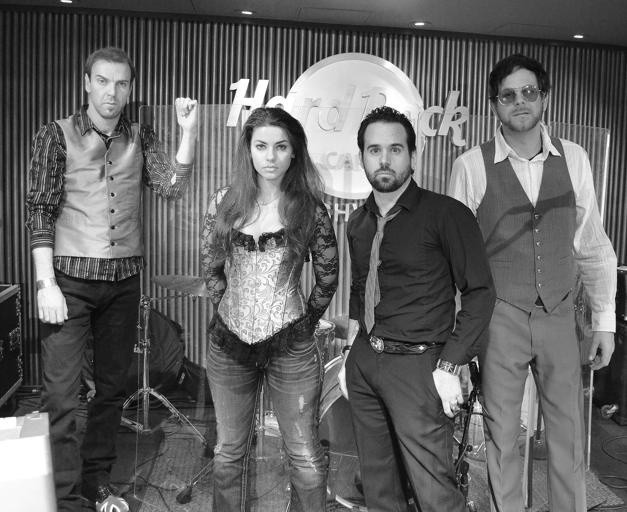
[360,330,443,354]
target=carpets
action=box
[140,406,624,511]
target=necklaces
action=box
[250,190,282,206]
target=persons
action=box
[200,106,340,512]
[28,46,199,512]
[447,54,617,512]
[338,108,498,511]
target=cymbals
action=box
[152,275,211,298]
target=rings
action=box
[450,399,459,407]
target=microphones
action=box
[468,361,480,394]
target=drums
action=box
[280,353,414,511]
[329,316,350,360]
[257,318,336,436]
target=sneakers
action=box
[82,481,130,511]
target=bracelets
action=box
[35,277,58,291]
[342,345,352,357]
[436,358,462,376]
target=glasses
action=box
[489,84,542,107]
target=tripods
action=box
[175,374,285,504]
[120,294,216,460]
